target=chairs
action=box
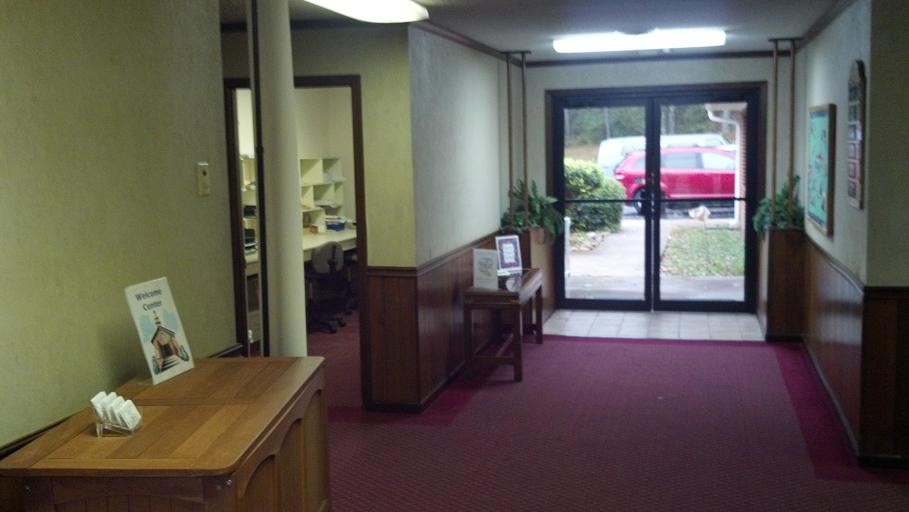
[305,242,353,333]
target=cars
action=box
[598,134,736,214]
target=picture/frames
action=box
[807,102,835,237]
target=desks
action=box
[245,227,357,278]
[462,267,543,382]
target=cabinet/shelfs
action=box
[0,355,331,511]
[240,156,347,241]
